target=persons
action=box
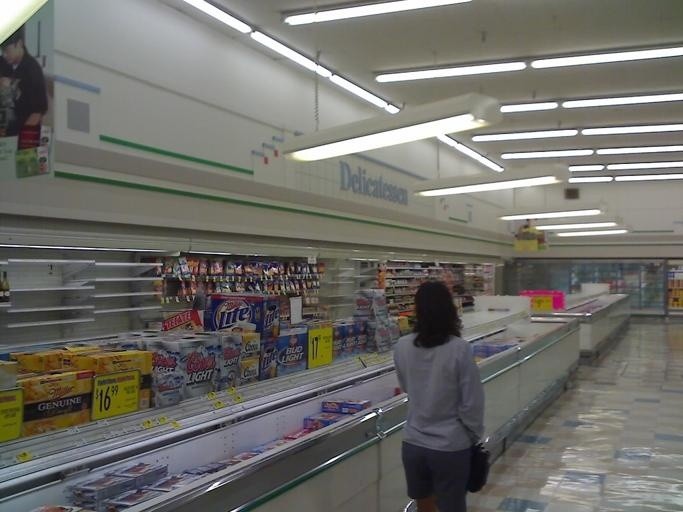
[41,137,49,146]
[394,279,484,512]
[452,285,475,312]
[1,24,48,139]
[39,157,48,173]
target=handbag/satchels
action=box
[463,437,493,493]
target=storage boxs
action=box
[108,290,401,420]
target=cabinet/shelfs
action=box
[382,261,484,315]
[0,243,169,352]
[347,253,383,313]
[320,263,352,314]
